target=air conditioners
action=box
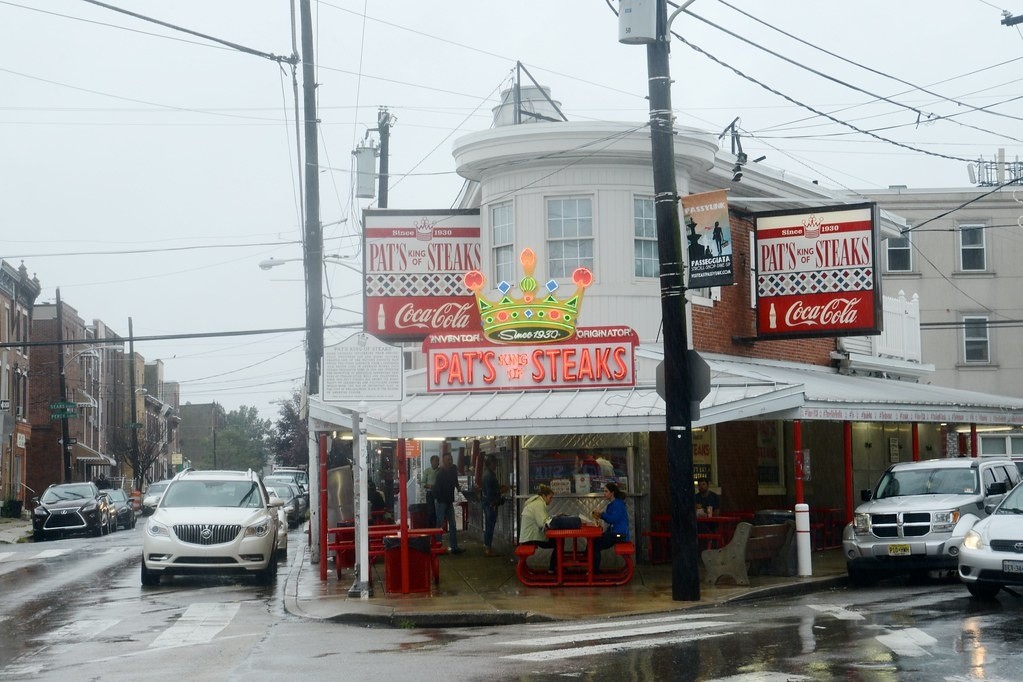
[15,406,23,416]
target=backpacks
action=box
[544,514,581,531]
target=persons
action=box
[694,476,719,508]
[421,452,467,555]
[519,485,565,575]
[368,481,385,511]
[585,481,630,576]
[481,455,509,557]
[576,449,615,491]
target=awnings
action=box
[73,388,97,408]
[76,442,104,461]
[86,454,116,467]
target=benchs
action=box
[368,548,447,557]
[815,519,846,526]
[641,531,722,540]
[327,538,382,546]
[613,540,635,556]
[369,541,443,551]
[513,543,537,556]
[328,543,382,552]
[809,520,826,529]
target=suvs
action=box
[138,466,284,586]
[842,455,1023,585]
[29,480,109,542]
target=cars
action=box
[260,466,309,529]
[141,480,172,516]
[97,485,137,532]
[957,476,1023,601]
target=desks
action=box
[515,522,634,589]
[367,527,447,589]
[647,511,756,566]
[767,506,845,552]
[328,523,410,580]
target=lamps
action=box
[338,431,398,442]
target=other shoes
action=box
[547,570,557,576]
[451,548,466,554]
[484,549,500,558]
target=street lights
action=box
[131,388,148,491]
[57,344,125,481]
